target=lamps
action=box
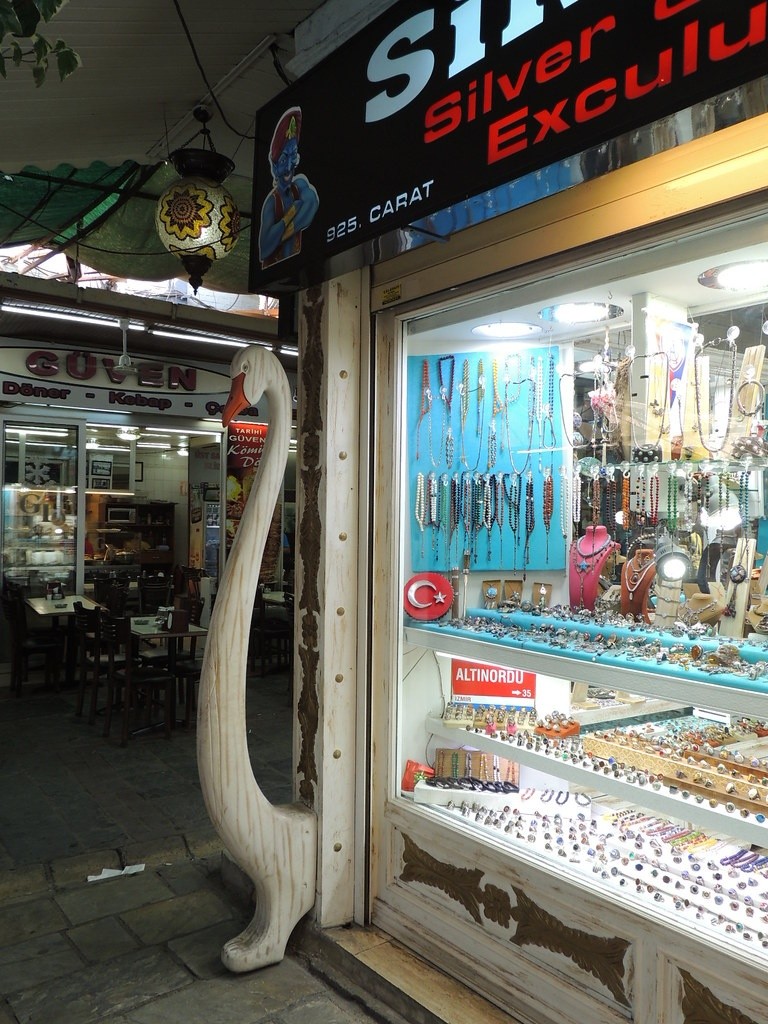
[154,102,240,291]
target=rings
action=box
[444,801,768,953]
[436,702,768,824]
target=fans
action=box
[71,318,140,376]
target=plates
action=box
[96,528,121,533]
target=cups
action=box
[242,475,282,583]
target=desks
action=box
[118,617,208,731]
[24,593,110,692]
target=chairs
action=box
[74,600,141,725]
[94,562,293,678]
[100,625,175,747]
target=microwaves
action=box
[107,508,135,523]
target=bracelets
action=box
[425,776,590,806]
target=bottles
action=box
[207,504,220,514]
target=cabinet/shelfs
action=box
[389,612,768,1024]
[98,501,178,567]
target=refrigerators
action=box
[190,483,222,578]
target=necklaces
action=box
[414,342,765,628]
